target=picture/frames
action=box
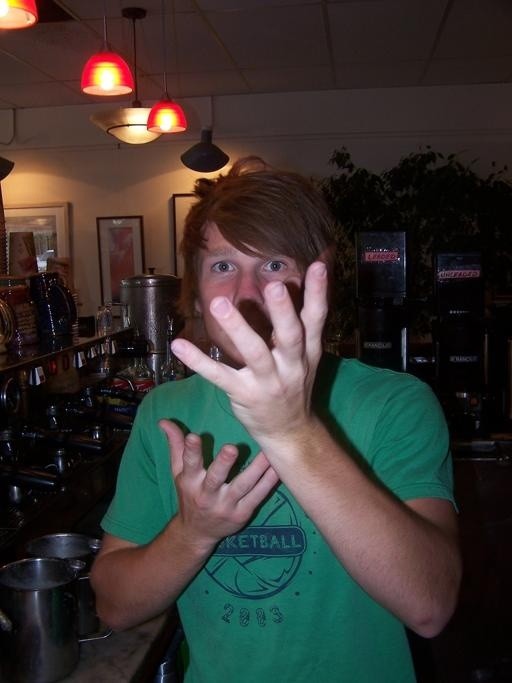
[172,193,203,277]
[3,201,72,287]
[96,215,145,319]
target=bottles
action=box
[127,329,185,392]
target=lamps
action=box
[79,16,136,98]
[178,126,229,175]
[147,1,188,134]
[88,7,164,146]
[0,1,40,31]
[2,155,16,179]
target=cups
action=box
[96,305,114,332]
[0,533,116,683]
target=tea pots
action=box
[28,272,76,340]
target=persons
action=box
[89,155,463,683]
